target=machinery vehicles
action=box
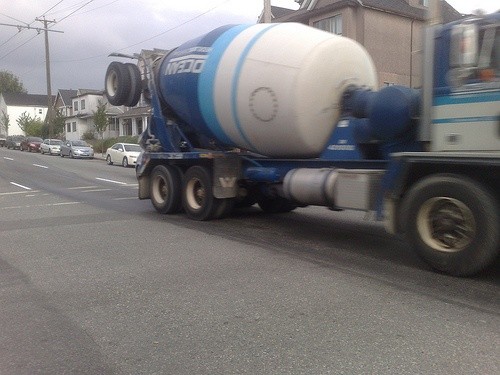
[105,9,499,275]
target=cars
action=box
[20,137,44,152]
[0,134,7,147]
[106,143,141,167]
[59,140,95,159]
[40,138,64,156]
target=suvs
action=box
[5,135,26,150]
